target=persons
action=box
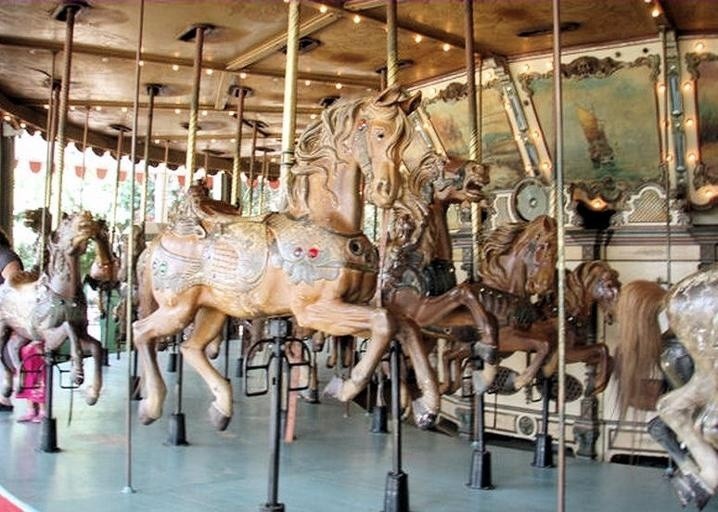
[16,319,48,423]
[0,229,31,288]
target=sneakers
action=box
[33,411,45,422]
[0,399,14,412]
[17,408,35,423]
